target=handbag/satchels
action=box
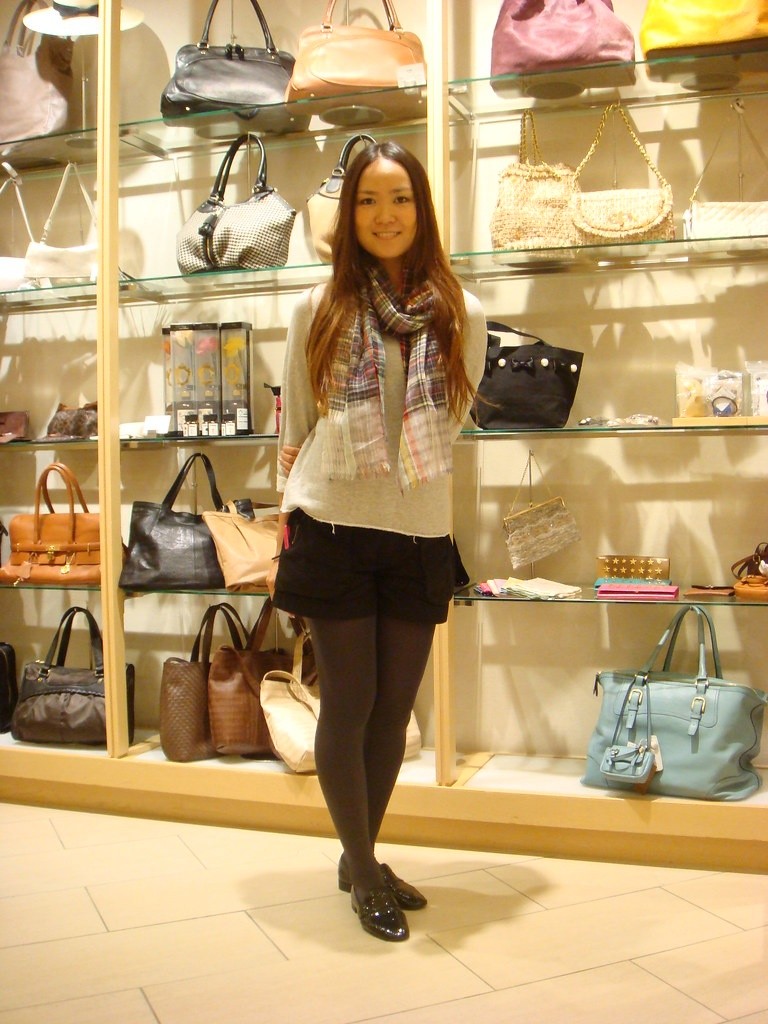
[158,603,252,762]
[0,463,129,588]
[504,451,580,570]
[207,596,315,755]
[0,0,767,446]
[10,606,134,745]
[731,542,768,580]
[0,641,19,733]
[734,574,768,603]
[118,452,256,591]
[600,677,654,783]
[201,500,286,592]
[260,630,422,772]
[580,605,768,800]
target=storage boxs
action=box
[162,327,177,437]
[219,322,256,435]
[168,323,198,436]
[193,322,221,434]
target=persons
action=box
[266,140,490,938]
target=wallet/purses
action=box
[594,577,671,590]
[597,584,680,602]
[684,583,735,597]
[596,555,671,579]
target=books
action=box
[474,577,523,596]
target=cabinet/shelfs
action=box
[0,0,768,850]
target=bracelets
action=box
[272,555,280,560]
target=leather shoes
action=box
[351,882,410,941]
[338,852,427,909]
